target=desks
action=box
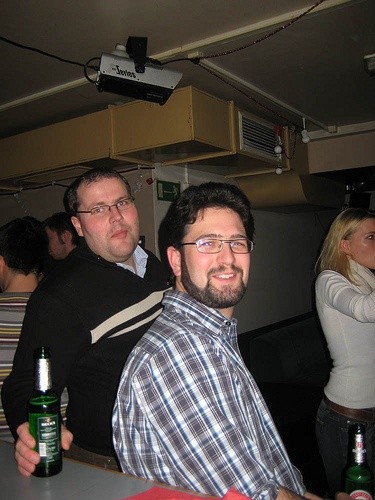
[0,438,220,500]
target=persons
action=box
[0,216,69,445]
[0,167,173,477]
[42,211,81,259]
[111,181,321,500]
[315,207,375,500]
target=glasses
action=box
[76,196,135,216]
[178,237,256,254]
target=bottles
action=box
[28,346,62,477]
[342,423,374,500]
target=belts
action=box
[322,393,375,422]
